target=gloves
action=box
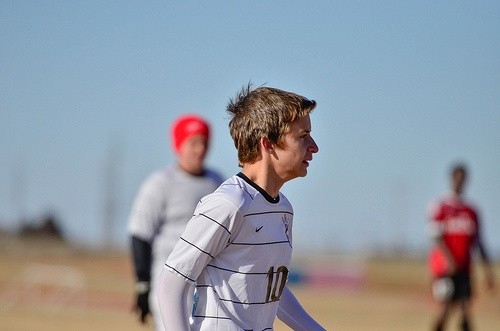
[136,291,149,322]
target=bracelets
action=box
[134,283,150,293]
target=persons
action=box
[155,82,328,331]
[127,116,226,325]
[428,164,494,331]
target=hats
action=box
[172,115,208,151]
